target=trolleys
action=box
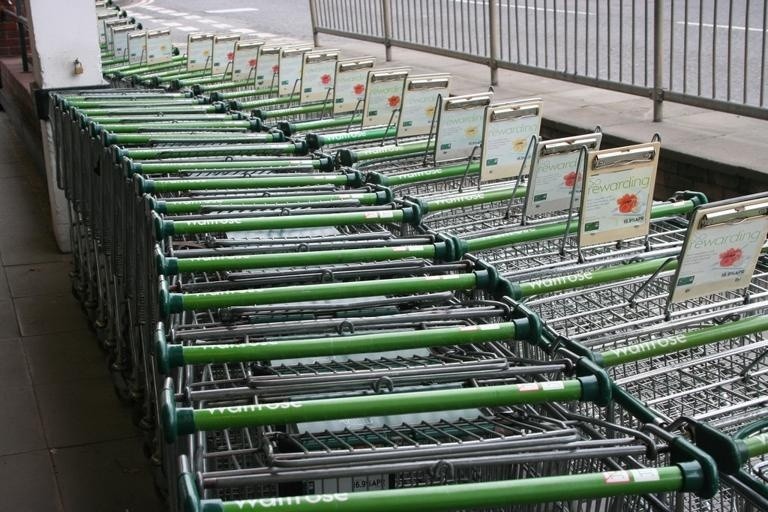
[46,1,768,512]
